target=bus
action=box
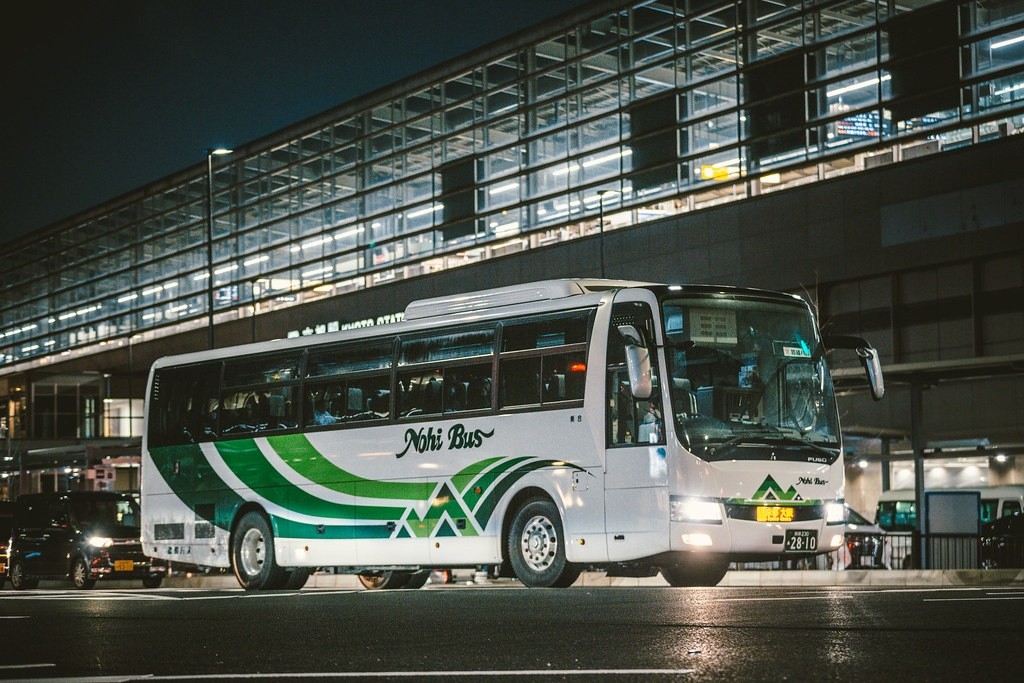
[873,484,1024,570]
[139,276,885,591]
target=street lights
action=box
[597,189,617,278]
[206,147,234,347]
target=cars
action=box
[842,505,885,568]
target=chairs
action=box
[267,380,445,428]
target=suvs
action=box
[7,490,169,591]
